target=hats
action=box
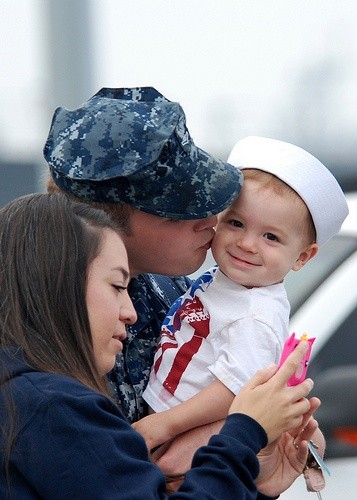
[227,137,349,248]
[43,87,242,220]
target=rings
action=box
[294,440,300,449]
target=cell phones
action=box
[279,332,316,387]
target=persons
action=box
[129,134,350,454]
[47,86,244,480]
[1,194,313,500]
